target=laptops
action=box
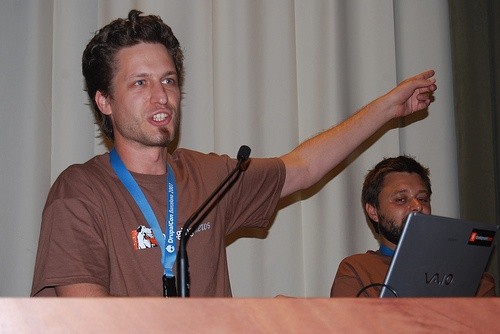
[379,211,500,298]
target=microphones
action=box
[177,146,251,297]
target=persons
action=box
[330,155,496,299]
[28,10,436,296]
